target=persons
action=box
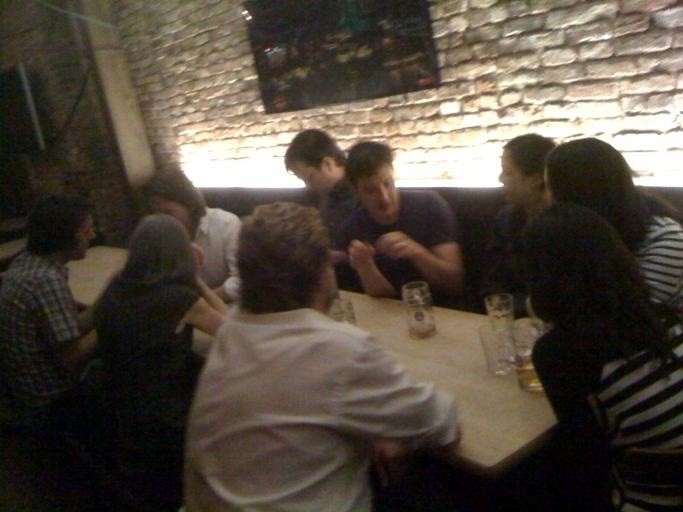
[493,134,556,318]
[544,138,683,309]
[285,129,361,291]
[520,204,683,512]
[184,202,461,512]
[0,197,99,436]
[96,215,230,512]
[336,142,464,298]
[142,168,243,302]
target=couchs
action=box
[193,188,683,314]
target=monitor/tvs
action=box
[1,62,50,154]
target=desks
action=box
[55,246,562,471]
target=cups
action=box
[326,298,355,325]
[401,280,437,340]
[509,317,548,392]
[477,323,515,378]
[484,292,513,325]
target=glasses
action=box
[358,177,395,194]
